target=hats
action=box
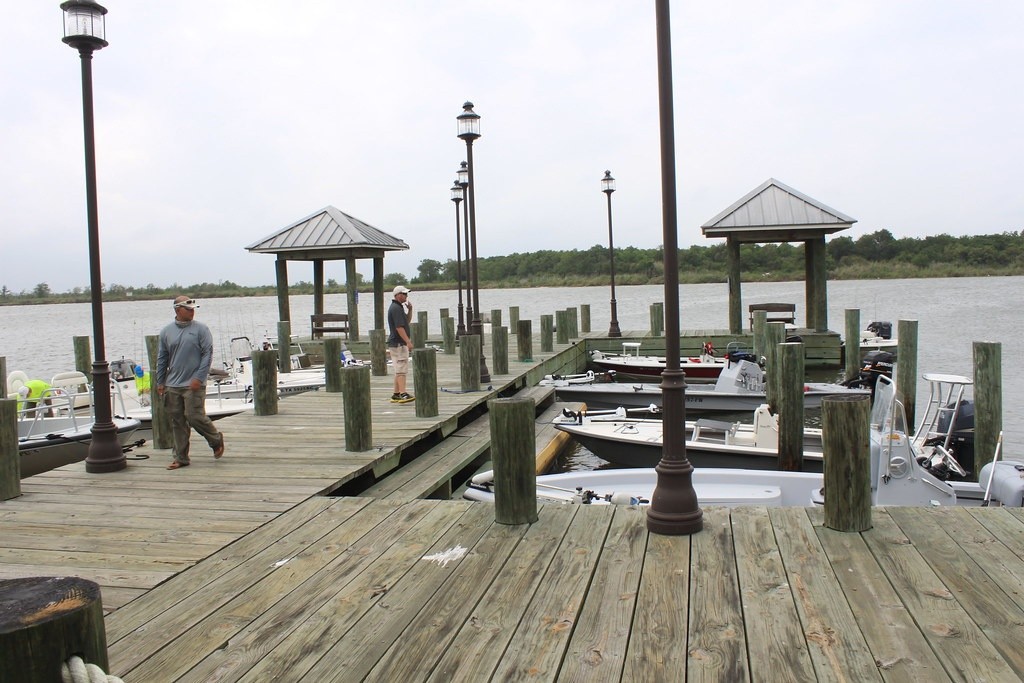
[393,285,411,297]
[173,299,200,310]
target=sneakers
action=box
[214,432,224,459]
[167,461,190,470]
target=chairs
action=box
[297,354,311,369]
[622,343,641,362]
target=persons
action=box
[134,366,150,395]
[386,286,415,404]
[156,296,225,470]
[17,379,53,418]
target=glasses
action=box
[176,300,195,305]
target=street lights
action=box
[449,101,491,379]
[59,0,128,474]
[600,169,623,337]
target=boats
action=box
[5,335,377,481]
[461,340,1024,507]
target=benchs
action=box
[51,371,93,416]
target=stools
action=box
[690,418,733,446]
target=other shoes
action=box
[390,393,401,403]
[398,393,415,403]
